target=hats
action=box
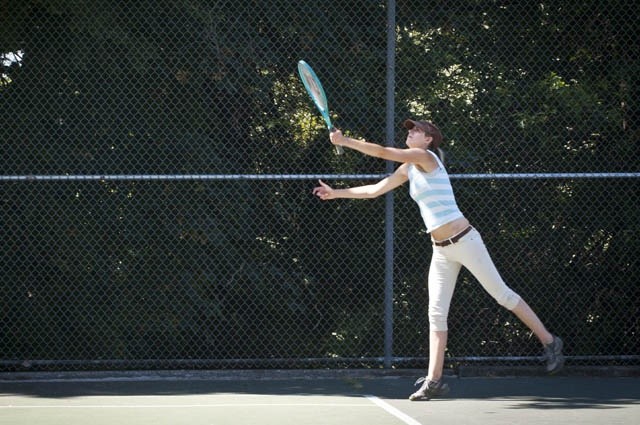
[405,120,443,149]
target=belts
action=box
[431,227,473,248]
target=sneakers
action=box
[543,336,565,374]
[409,376,451,400]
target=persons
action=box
[311,118,565,401]
